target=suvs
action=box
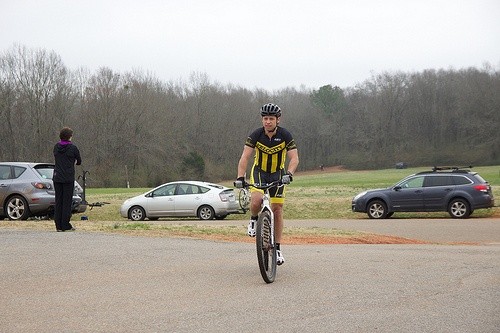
[0,161,87,221]
[351,165,495,219]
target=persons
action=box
[237,102,299,265]
[53,127,82,232]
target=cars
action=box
[120,180,239,221]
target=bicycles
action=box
[239,179,253,212]
[233,176,294,284]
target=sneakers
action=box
[247,219,257,237]
[275,250,284,264]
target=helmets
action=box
[260,103,281,117]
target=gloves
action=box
[282,174,291,185]
[235,177,245,188]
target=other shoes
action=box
[56,226,62,231]
[64,227,76,232]
